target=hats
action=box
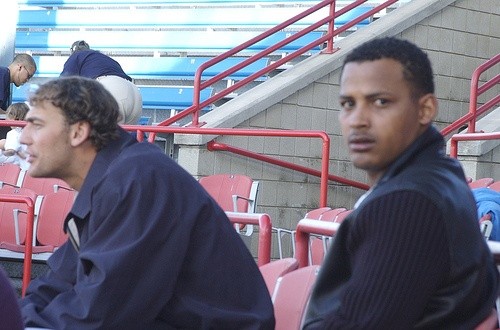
[70,40,89,52]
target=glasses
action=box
[24,66,32,81]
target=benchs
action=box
[9,9,374,161]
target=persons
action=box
[20,75,277,330]
[299,38,500,330]
[0,40,143,175]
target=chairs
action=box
[196,174,260,237]
[0,162,77,265]
[258,177,500,330]
